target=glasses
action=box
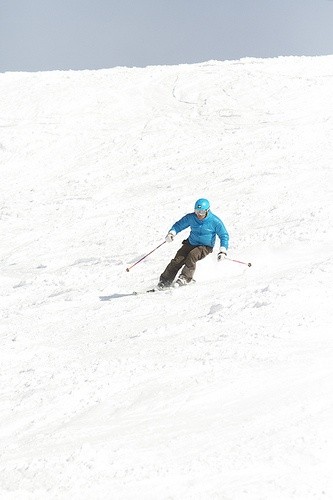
[195,210,207,216]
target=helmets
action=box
[194,198,210,211]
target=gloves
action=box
[217,253,225,262]
[165,232,174,242]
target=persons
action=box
[156,199,229,287]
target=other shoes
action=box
[174,274,191,288]
[153,281,171,290]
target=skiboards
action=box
[132,275,196,294]
